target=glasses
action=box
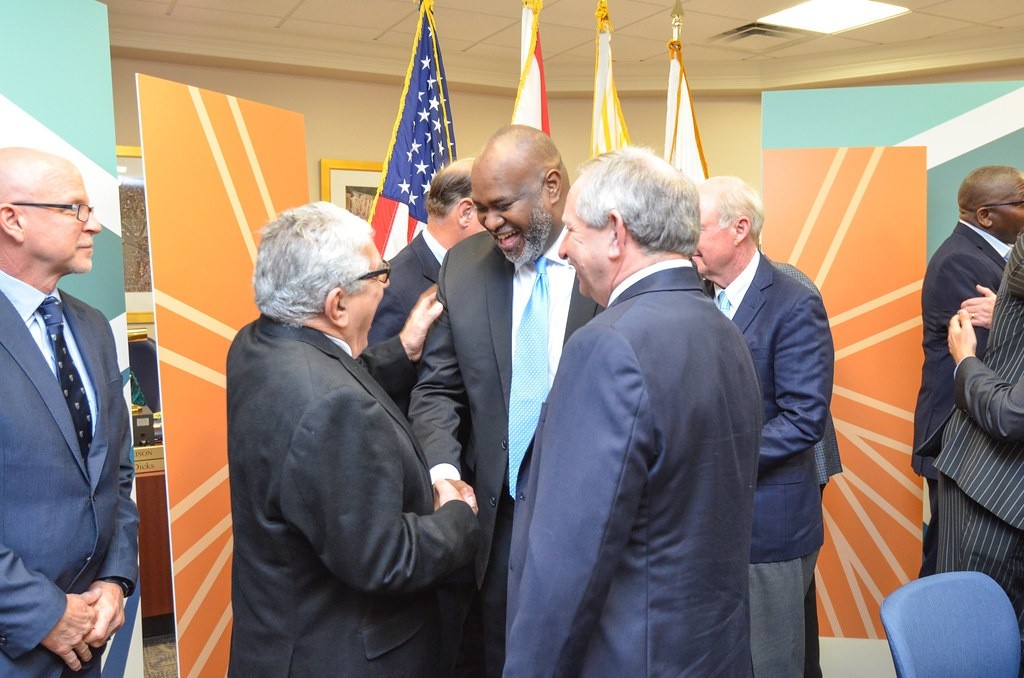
[358,259,392,284]
[9,202,95,223]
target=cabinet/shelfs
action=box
[135,470,176,639]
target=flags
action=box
[511,0,550,137]
[591,25,631,159]
[663,43,707,181]
[366,0,459,260]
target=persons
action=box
[910,165,1024,650]
[225,202,483,678]
[0,147,140,678]
[366,125,844,678]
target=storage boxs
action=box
[134,445,164,460]
[134,459,166,473]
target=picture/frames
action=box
[320,158,386,222]
[115,144,154,324]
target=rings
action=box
[972,313,974,318]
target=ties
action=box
[717,291,732,319]
[507,256,550,502]
[36,295,93,472]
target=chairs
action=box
[879,570,1022,678]
